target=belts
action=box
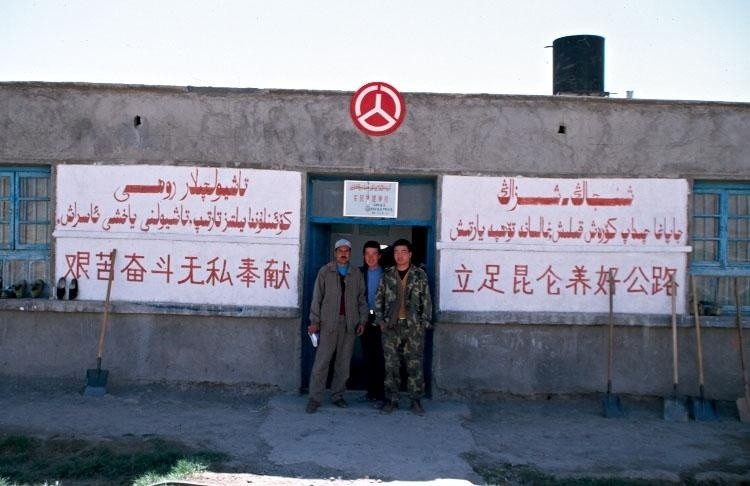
[368,309,375,315]
[396,319,408,325]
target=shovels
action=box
[599,268,750,423]
[81,249,116,395]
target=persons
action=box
[303,238,369,416]
[325,240,389,411]
[372,239,434,418]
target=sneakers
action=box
[305,397,320,414]
[332,398,349,408]
[373,397,398,415]
[410,398,426,415]
[356,393,376,403]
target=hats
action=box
[335,239,352,250]
